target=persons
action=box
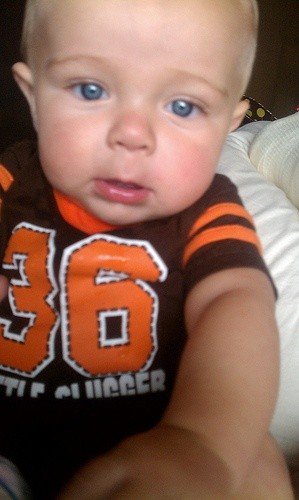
[0,0,294,500]
